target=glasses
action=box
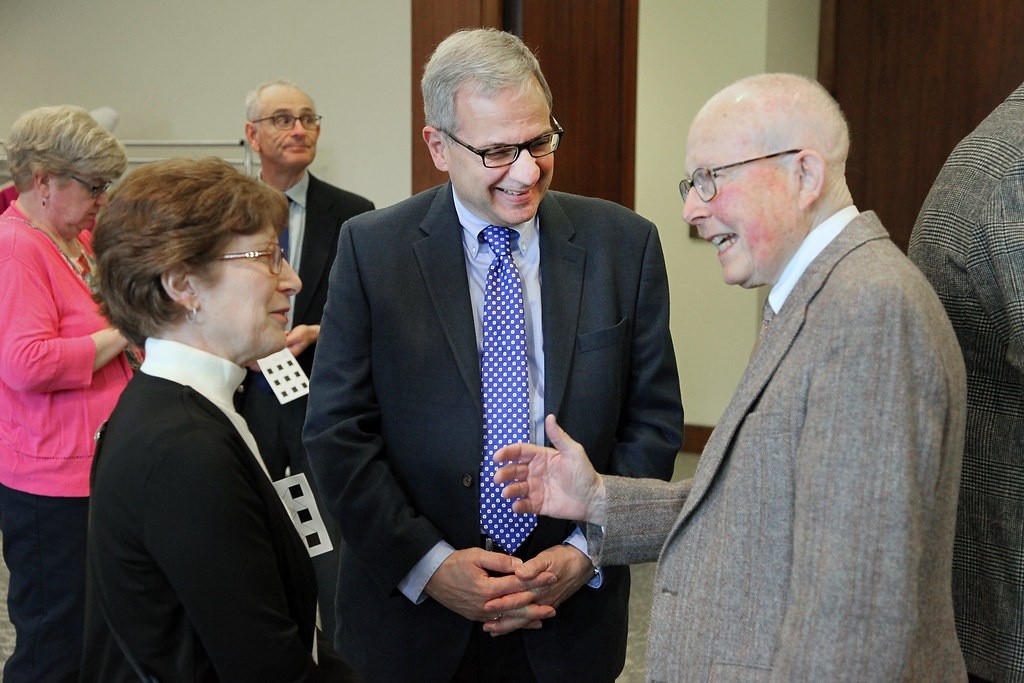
[436,114,566,168]
[209,241,285,275]
[250,113,323,130]
[68,174,113,199]
[679,149,802,202]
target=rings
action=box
[490,612,503,621]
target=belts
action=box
[482,538,523,562]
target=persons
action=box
[909,84,1024,683]
[302,28,683,683]
[0,78,377,683]
[493,73,968,683]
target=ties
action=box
[477,223,540,557]
[278,194,295,264]
[757,294,775,334]
